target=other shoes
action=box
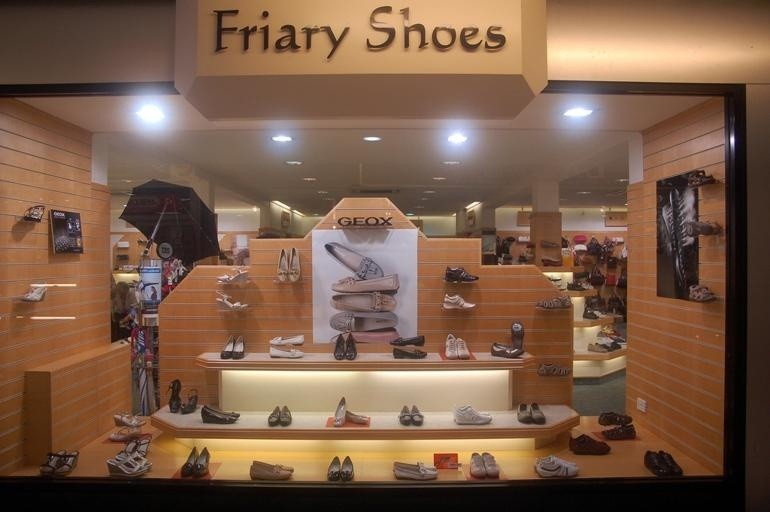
[393,461,438,479]
[279,406,292,426]
[345,333,357,359]
[517,402,529,422]
[269,335,305,358]
[333,397,346,427]
[253,460,294,473]
[529,402,545,423]
[250,465,291,480]
[341,456,354,482]
[193,447,210,477]
[345,410,370,425]
[570,410,639,457]
[644,449,684,480]
[334,333,346,361]
[268,406,281,427]
[181,447,199,476]
[400,406,411,426]
[410,405,424,426]
[327,457,341,482]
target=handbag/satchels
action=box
[580,238,627,315]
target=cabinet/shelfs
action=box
[540,265,626,386]
[151,196,580,440]
[12,215,45,304]
[685,180,722,303]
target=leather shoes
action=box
[587,334,625,353]
[583,308,599,319]
[288,247,301,282]
[325,239,401,345]
[276,248,288,282]
[507,238,594,291]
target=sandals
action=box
[214,289,249,311]
[166,378,181,413]
[23,203,46,223]
[687,169,715,188]
[181,389,197,414]
[214,268,251,286]
[536,362,571,377]
[537,294,573,309]
[20,281,48,302]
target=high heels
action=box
[393,347,428,360]
[52,449,80,479]
[107,414,152,479]
[221,336,244,359]
[390,335,425,346]
[39,447,69,478]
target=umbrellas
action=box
[144,327,156,414]
[136,327,148,416]
[118,177,222,264]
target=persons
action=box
[111,280,140,326]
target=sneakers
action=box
[481,453,500,478]
[441,292,478,311]
[491,342,523,358]
[511,323,524,350]
[444,265,480,283]
[689,284,719,303]
[535,457,580,478]
[683,220,720,236]
[454,404,493,425]
[445,333,458,360]
[456,337,469,359]
[470,453,487,478]
